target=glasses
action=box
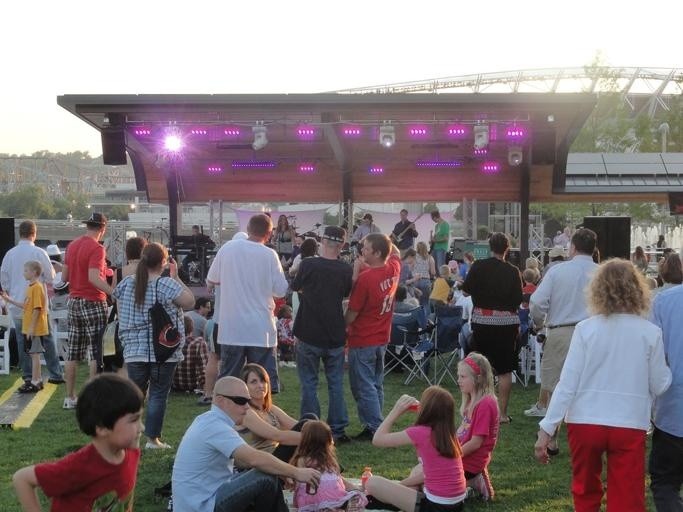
[224,395,250,405]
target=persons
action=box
[284,421,369,512]
[171,376,322,512]
[199,231,249,404]
[233,363,344,473]
[523,229,604,418]
[364,386,467,512]
[0,209,682,396]
[289,227,354,444]
[533,256,673,512]
[463,235,523,424]
[410,352,500,501]
[61,212,117,409]
[11,372,145,512]
[344,234,402,439]
[649,283,683,512]
[111,242,197,447]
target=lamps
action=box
[164,121,182,151]
[378,120,396,148]
[250,120,268,151]
[507,142,523,168]
[473,121,489,150]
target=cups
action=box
[408,401,420,411]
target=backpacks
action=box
[148,276,180,383]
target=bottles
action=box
[362,467,373,489]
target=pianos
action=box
[165,244,209,248]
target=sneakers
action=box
[524,404,547,417]
[18,378,78,410]
[146,441,172,449]
[476,467,494,500]
[334,426,373,445]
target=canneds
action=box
[305,479,317,495]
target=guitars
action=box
[429,230,434,253]
[392,213,424,247]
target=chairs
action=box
[521,328,546,387]
[382,305,426,385]
[405,303,463,389]
[40,310,69,367]
[0,315,11,375]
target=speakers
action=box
[583,215,631,262]
[0,216,15,267]
[101,128,127,165]
[453,239,466,261]
[531,129,557,165]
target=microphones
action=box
[201,225,204,232]
[286,215,295,218]
[314,223,321,226]
[370,219,373,224]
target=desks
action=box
[630,250,663,274]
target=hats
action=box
[323,225,346,242]
[81,212,106,226]
[47,244,61,256]
[52,272,70,291]
